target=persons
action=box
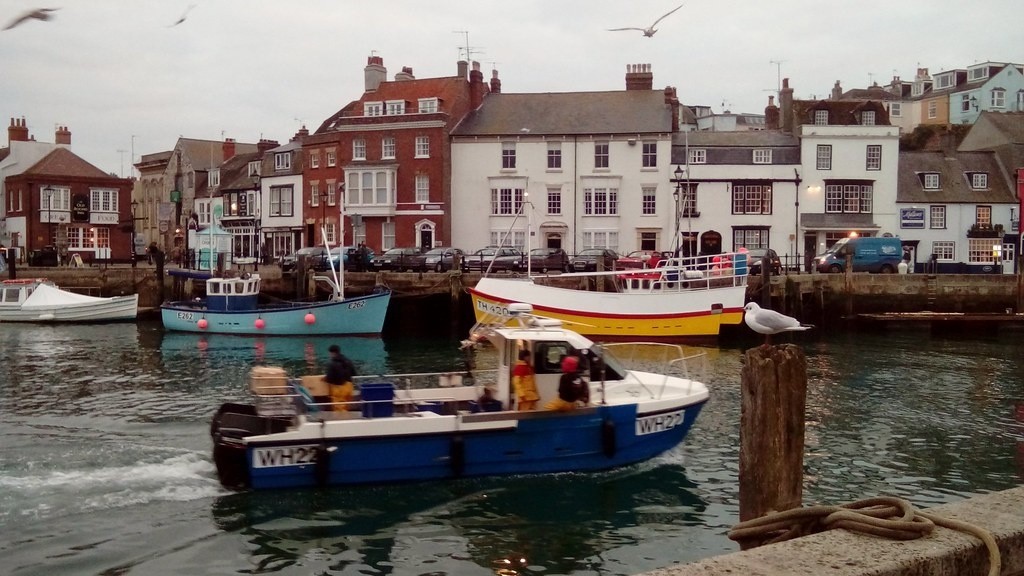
[321,345,359,412]
[535,344,564,374]
[542,357,589,412]
[259,242,265,265]
[477,384,503,412]
[149,241,159,264]
[898,251,914,274]
[511,350,541,412]
[356,241,367,272]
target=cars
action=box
[461,249,521,273]
[325,246,379,273]
[565,249,618,273]
[746,248,782,276]
[513,248,568,274]
[369,247,427,273]
[278,248,328,272]
[420,247,465,273]
[616,250,667,279]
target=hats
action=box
[562,356,581,374]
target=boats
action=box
[210,301,708,495]
[160,191,392,335]
[465,201,749,347]
[0,277,139,323]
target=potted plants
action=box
[967,222,1003,238]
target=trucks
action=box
[812,237,902,274]
[134,233,147,257]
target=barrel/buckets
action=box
[733,254,746,275]
[415,401,448,415]
[898,263,907,274]
[468,399,502,413]
[359,383,393,417]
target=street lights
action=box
[131,199,139,266]
[44,184,55,251]
[319,190,329,247]
[674,165,685,251]
[250,170,260,271]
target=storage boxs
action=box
[251,367,296,417]
[469,399,503,413]
[411,400,444,416]
[358,383,392,417]
[302,375,330,398]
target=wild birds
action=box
[166,4,198,28]
[605,2,683,37]
[1,6,60,31]
[743,302,815,345]
